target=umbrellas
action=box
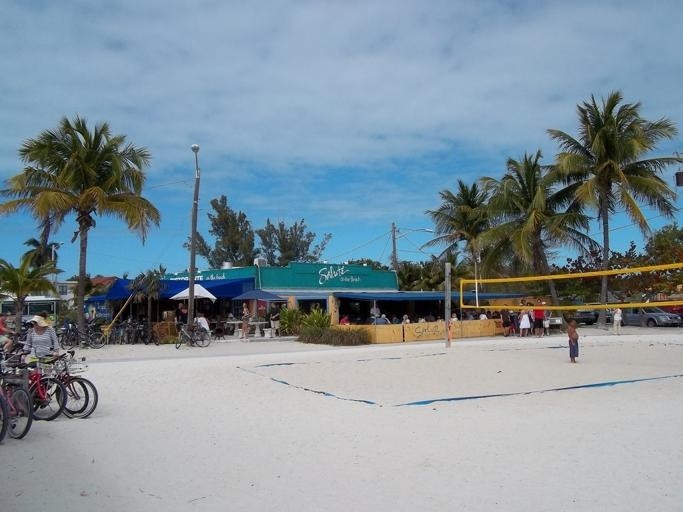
[169,284,217,317]
[231,289,288,320]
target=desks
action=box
[209,320,268,337]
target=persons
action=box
[340,298,552,338]
[0,314,15,350]
[194,313,211,336]
[613,308,622,335]
[567,319,578,363]
[270,303,281,337]
[175,303,188,324]
[240,302,249,340]
[22,312,59,354]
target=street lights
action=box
[187,144,200,323]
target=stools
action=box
[263,328,277,338]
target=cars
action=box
[564,302,683,327]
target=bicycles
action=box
[0,317,211,442]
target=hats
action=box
[27,316,47,327]
